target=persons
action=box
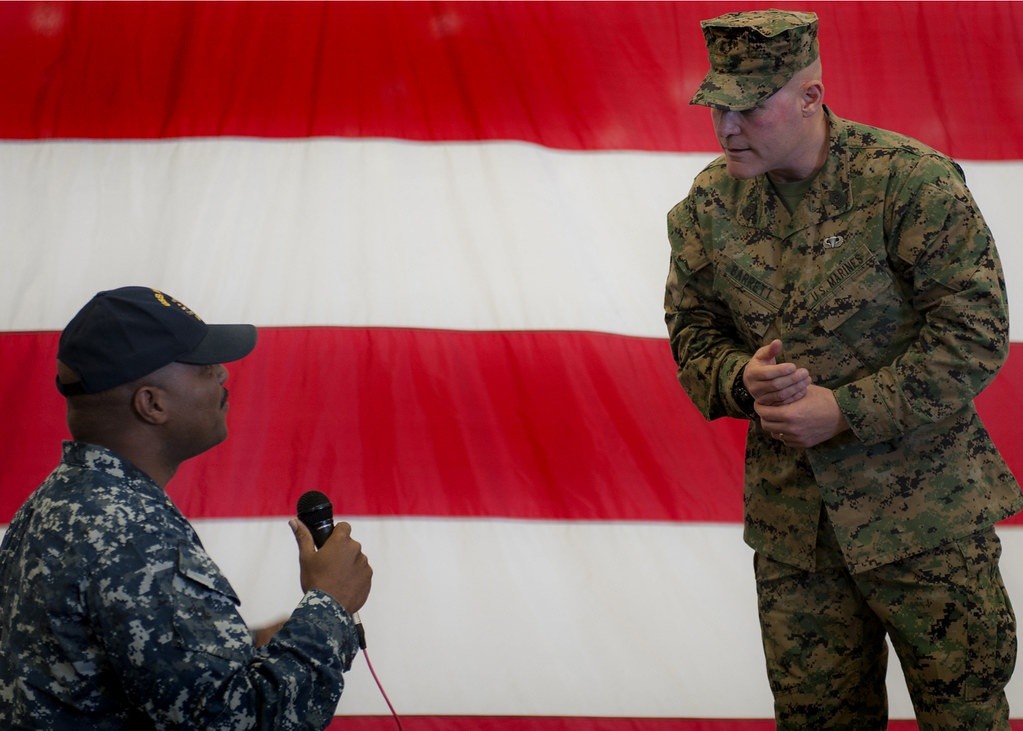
[1,288,373,731]
[663,10,1017,731]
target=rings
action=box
[779,433,783,441]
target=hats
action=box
[55,286,256,398]
[687,8,819,112]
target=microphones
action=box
[297,491,368,651]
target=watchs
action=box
[732,368,756,415]
